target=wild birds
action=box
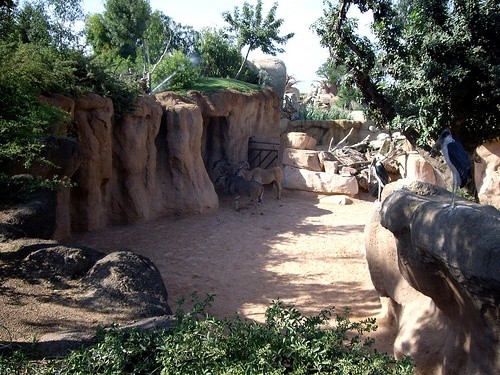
[370,155,389,201]
[430,128,472,214]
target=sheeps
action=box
[212,155,250,180]
[214,169,264,210]
[235,162,283,200]
